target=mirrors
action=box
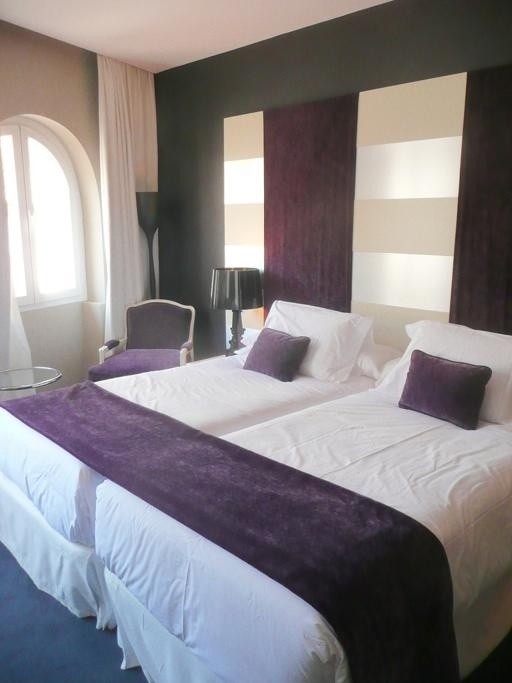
[222,112,267,271]
[348,69,469,324]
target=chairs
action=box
[86,299,197,381]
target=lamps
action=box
[208,267,264,355]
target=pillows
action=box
[241,298,511,431]
